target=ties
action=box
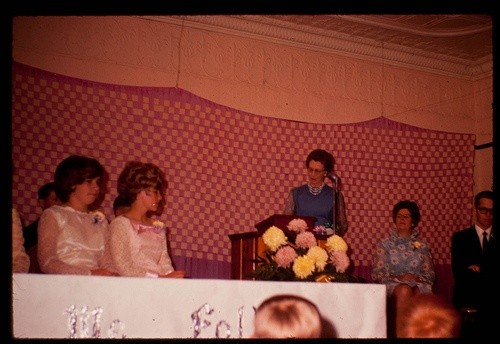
[482,232,489,252]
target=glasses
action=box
[308,167,326,174]
[477,207,493,215]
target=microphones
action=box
[327,172,337,181]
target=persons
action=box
[397,294,460,338]
[38,155,120,276]
[255,295,322,339]
[23,182,62,274]
[284,149,348,237]
[108,161,186,278]
[451,190,500,344]
[12,207,31,273]
[372,200,436,309]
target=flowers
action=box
[92,212,105,224]
[413,241,420,251]
[153,221,164,232]
[248,218,349,278]
